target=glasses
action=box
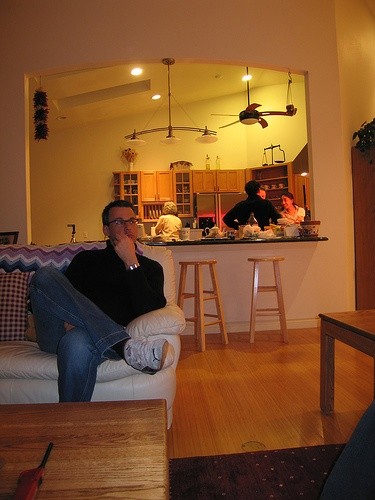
[106,218,138,225]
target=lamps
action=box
[124,57,218,145]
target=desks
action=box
[318,311,375,411]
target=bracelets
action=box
[128,263,140,270]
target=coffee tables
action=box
[0,399,169,500]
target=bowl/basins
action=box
[189,229,203,240]
[300,221,321,237]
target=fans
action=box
[211,67,288,129]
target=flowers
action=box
[120,146,137,162]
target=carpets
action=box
[170,443,345,500]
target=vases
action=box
[128,162,133,171]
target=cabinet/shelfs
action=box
[113,172,139,220]
[173,171,192,217]
[249,161,295,215]
[141,171,174,201]
[192,171,242,193]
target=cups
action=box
[239,225,245,238]
[180,227,190,240]
[151,227,156,237]
[264,226,270,231]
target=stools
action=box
[177,260,228,350]
[247,257,289,345]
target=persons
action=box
[280,192,305,223]
[155,202,182,240]
[258,188,266,199]
[223,181,282,231]
[30,200,167,403]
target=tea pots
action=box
[205,224,225,238]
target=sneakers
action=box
[124,337,169,375]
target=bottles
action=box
[206,155,210,169]
[249,213,258,227]
[216,156,220,169]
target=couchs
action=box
[0,242,186,430]
